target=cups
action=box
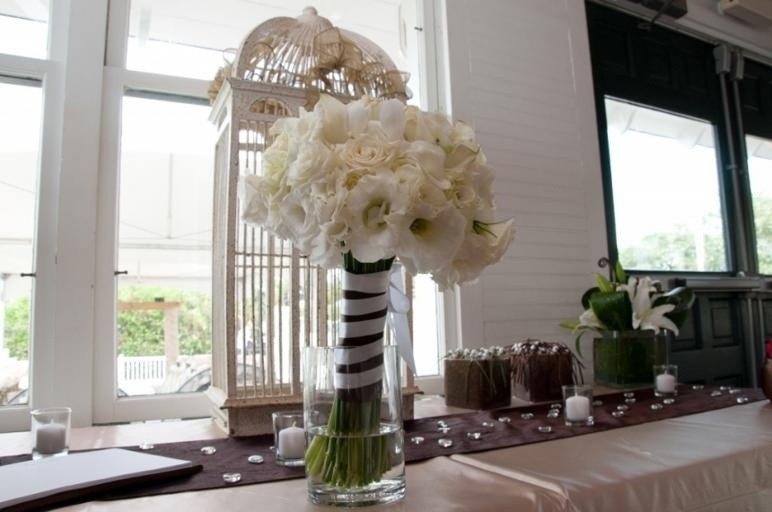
[562,385,595,426]
[653,364,678,398]
[272,410,308,467]
[30,407,73,461]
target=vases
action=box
[592,326,672,390]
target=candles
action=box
[37,418,65,453]
[657,369,675,393]
[565,392,590,421]
[277,420,308,457]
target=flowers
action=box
[238,91,520,487]
[559,257,698,382]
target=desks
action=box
[0,385,771,511]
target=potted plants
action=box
[443,340,587,410]
